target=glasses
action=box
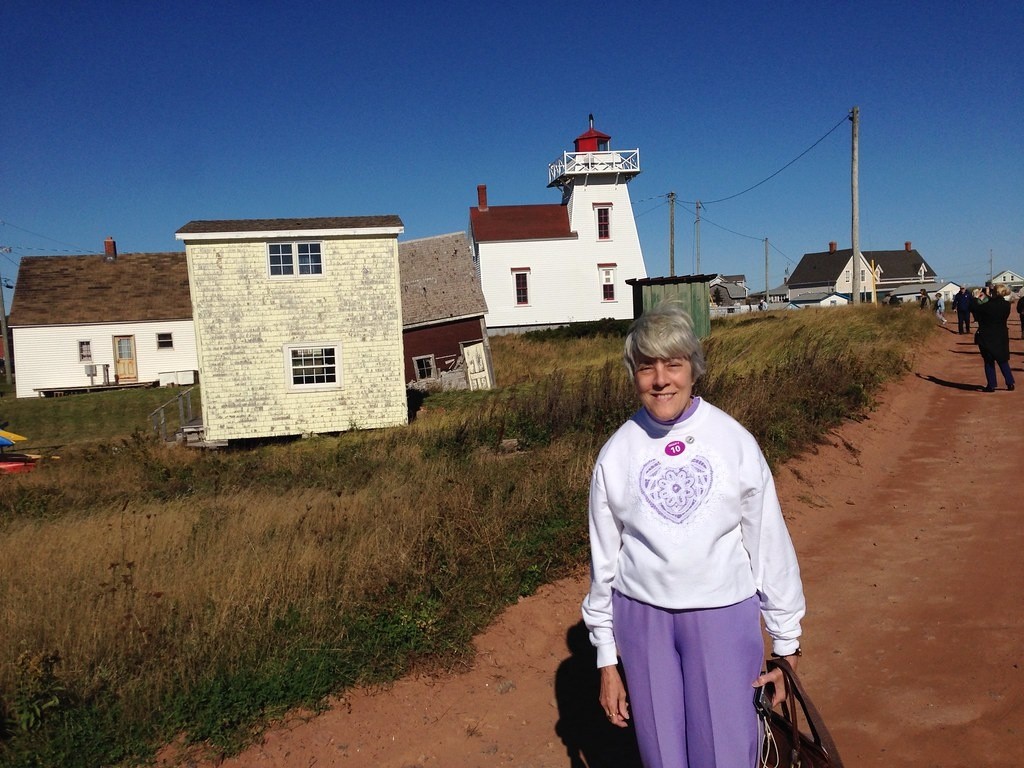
[961,288,964,290]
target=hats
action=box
[960,285,965,288]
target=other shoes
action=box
[943,320,947,324]
[1008,385,1014,390]
[982,385,995,392]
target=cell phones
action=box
[982,288,986,293]
[754,671,775,716]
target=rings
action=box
[609,714,615,716]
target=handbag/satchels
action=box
[974,329,979,345]
[759,658,844,768]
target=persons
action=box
[920,289,931,310]
[581,307,806,768]
[935,293,947,324]
[760,300,768,311]
[951,283,1024,392]
